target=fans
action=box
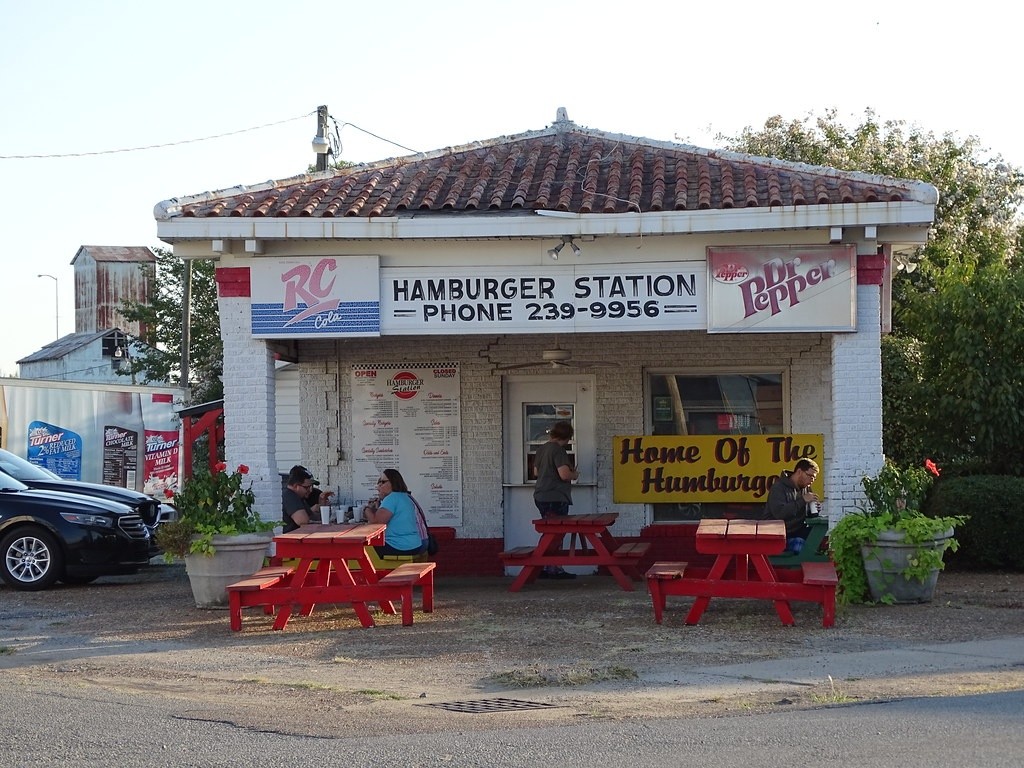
[500,333,619,374]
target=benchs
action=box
[383,551,430,570]
[793,556,845,623]
[768,549,796,567]
[497,546,538,559]
[379,560,440,627]
[615,541,652,559]
[226,564,296,630]
[642,559,694,626]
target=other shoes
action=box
[542,569,577,580]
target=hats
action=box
[289,465,320,486]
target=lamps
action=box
[547,234,583,260]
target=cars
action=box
[0,447,165,562]
[0,471,150,591]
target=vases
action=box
[856,524,955,603]
[184,533,271,611]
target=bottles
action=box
[142,393,180,496]
[4,385,144,492]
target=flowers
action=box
[833,445,978,617]
[150,453,286,563]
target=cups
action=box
[319,506,329,525]
[360,504,365,518]
[340,505,350,513]
[331,505,339,518]
[335,510,344,524]
[353,507,361,522]
[809,501,818,513]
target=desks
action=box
[804,515,829,560]
[682,518,793,627]
[508,511,638,593]
[271,520,391,632]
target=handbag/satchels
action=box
[428,534,439,556]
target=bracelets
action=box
[363,505,370,512]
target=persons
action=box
[760,458,822,554]
[533,422,580,579]
[282,466,335,535]
[363,469,429,559]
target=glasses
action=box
[378,479,390,484]
[298,484,313,490]
[805,472,816,481]
